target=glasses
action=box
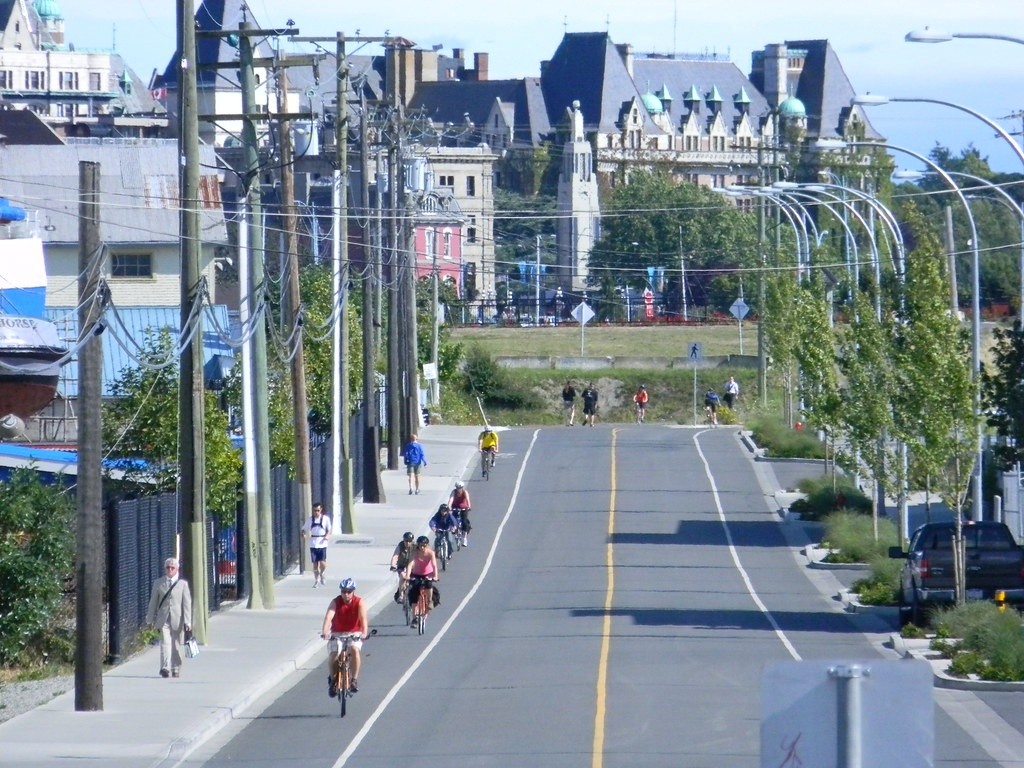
[314,510,320,512]
[342,590,353,594]
[165,566,175,570]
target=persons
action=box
[563,382,576,425]
[404,434,427,494]
[582,383,598,426]
[148,559,191,678]
[448,482,470,546]
[725,376,738,409]
[323,579,367,697]
[478,425,498,477]
[429,504,457,559]
[391,532,437,628]
[302,503,331,587]
[634,386,647,421]
[705,388,721,424]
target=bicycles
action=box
[450,507,471,551]
[705,403,720,429]
[321,633,371,718]
[403,575,441,635]
[635,401,645,424]
[389,567,414,626]
[434,528,455,571]
[480,450,498,481]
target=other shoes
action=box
[313,581,319,588]
[409,490,413,495]
[328,677,337,698]
[415,489,420,495]
[172,670,179,678]
[411,619,418,628]
[463,539,467,547]
[482,471,487,478]
[397,597,404,603]
[713,421,718,425]
[159,667,169,678]
[491,461,495,467]
[351,679,359,692]
[427,602,433,610]
[321,578,326,585]
[582,419,587,426]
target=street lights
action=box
[850,93,1024,163]
[814,139,984,520]
[712,170,908,545]
[623,242,640,322]
[534,233,557,328]
[892,171,1023,219]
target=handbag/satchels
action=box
[405,456,410,465]
[723,392,728,400]
[184,631,199,659]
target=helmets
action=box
[339,578,355,590]
[403,481,464,543]
[485,425,492,431]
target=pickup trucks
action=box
[888,520,1024,628]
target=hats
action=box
[640,385,645,389]
